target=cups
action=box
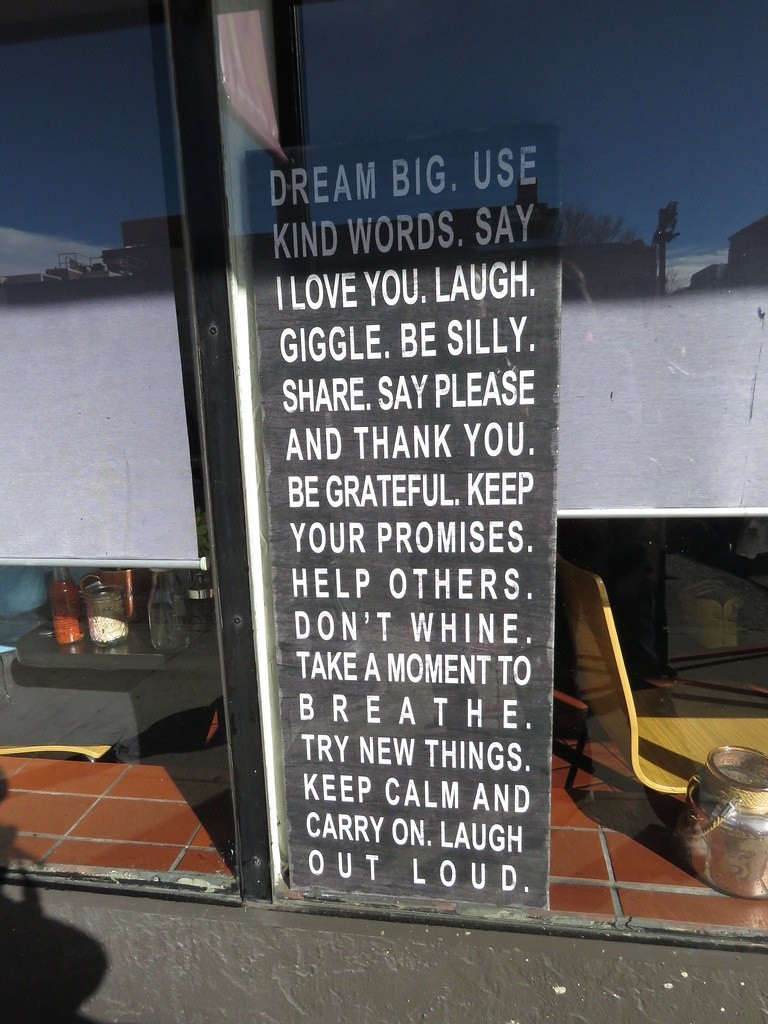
[79,566,141,622]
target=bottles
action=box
[188,569,215,633]
[84,584,129,648]
[147,568,189,651]
[681,746,767,901]
[50,567,85,644]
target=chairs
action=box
[557,557,768,795]
